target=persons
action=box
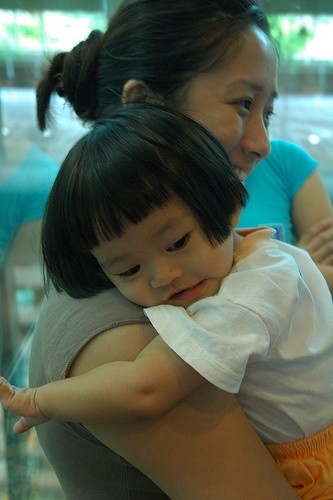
[231,138,333,289]
[22,0,278,500]
[1,101,333,500]
